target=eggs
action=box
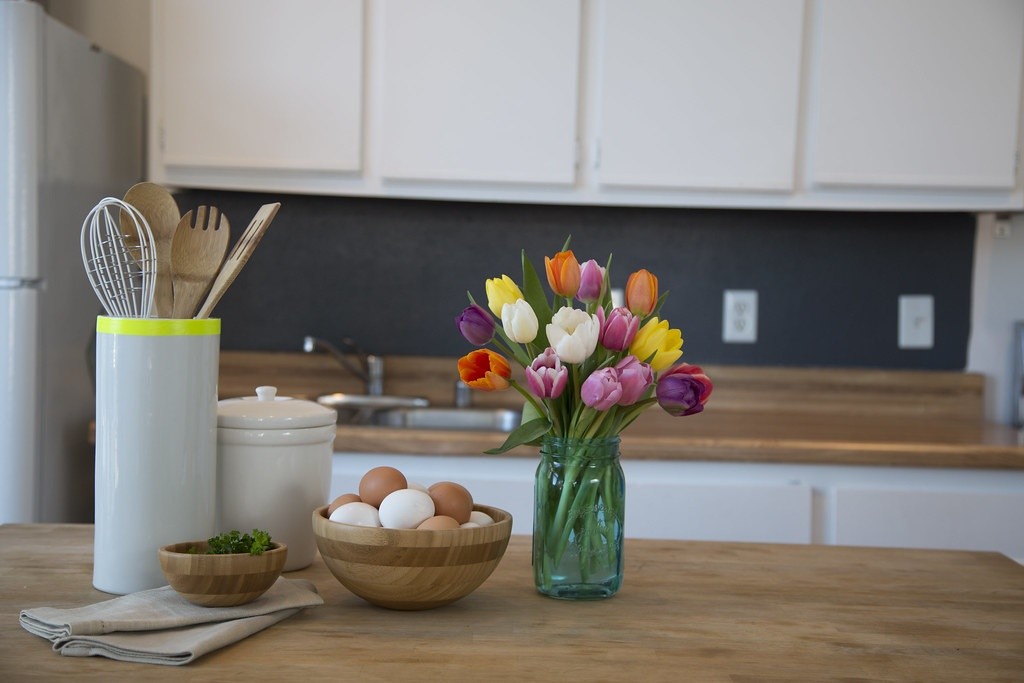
[328,465,495,530]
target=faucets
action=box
[303,335,433,408]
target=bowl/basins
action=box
[158,538,287,608]
[314,504,513,609]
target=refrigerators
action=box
[1,0,150,529]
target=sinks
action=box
[357,405,525,433]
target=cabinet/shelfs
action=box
[146,0,588,207]
[585,0,1024,213]
[620,458,1024,568]
[325,451,531,537]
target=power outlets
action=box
[896,292,935,351]
[611,288,625,309]
[721,288,758,345]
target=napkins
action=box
[51,579,317,666]
[18,574,326,642]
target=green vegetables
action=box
[185,527,273,556]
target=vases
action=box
[530,434,627,602]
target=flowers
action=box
[454,233,713,591]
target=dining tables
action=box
[0,517,1024,683]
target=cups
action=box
[215,388,338,573]
